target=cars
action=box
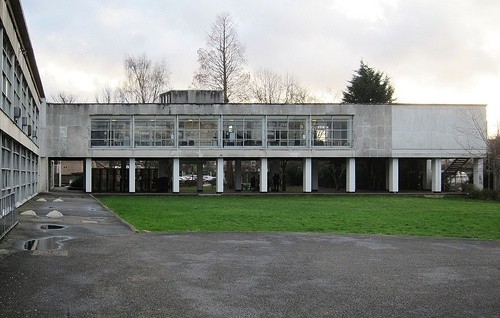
[179,175,228,185]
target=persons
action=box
[273,173,279,192]
